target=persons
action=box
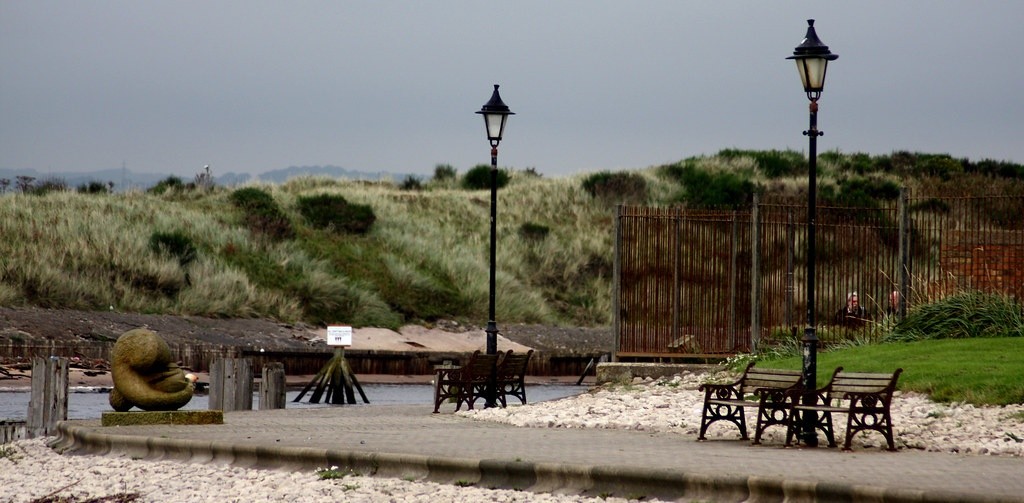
[886,291,900,317]
[831,292,873,331]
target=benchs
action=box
[697,362,814,446]
[470,349,534,410]
[785,366,904,451]
[432,350,504,413]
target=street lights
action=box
[471,84,517,410]
[783,17,839,453]
